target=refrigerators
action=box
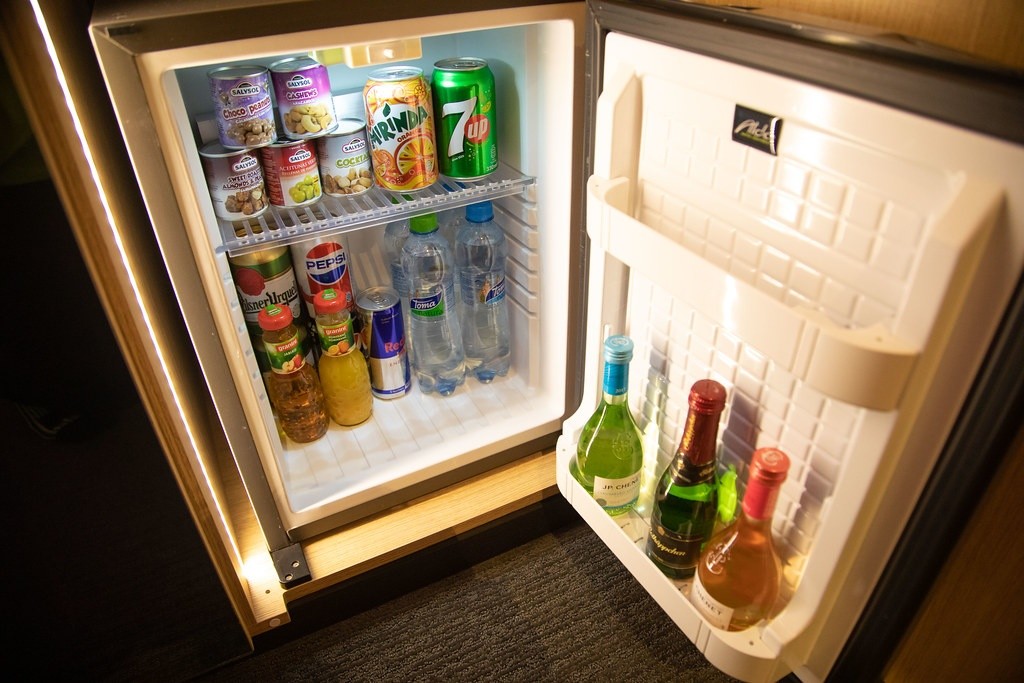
[0,0,1024,683]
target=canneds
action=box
[198,55,499,221]
[224,232,412,409]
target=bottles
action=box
[400,213,465,397]
[646,379,728,581]
[455,201,512,383]
[437,181,466,328]
[572,334,643,516]
[691,449,790,631]
[313,288,374,426]
[384,194,415,366]
[257,304,330,443]
